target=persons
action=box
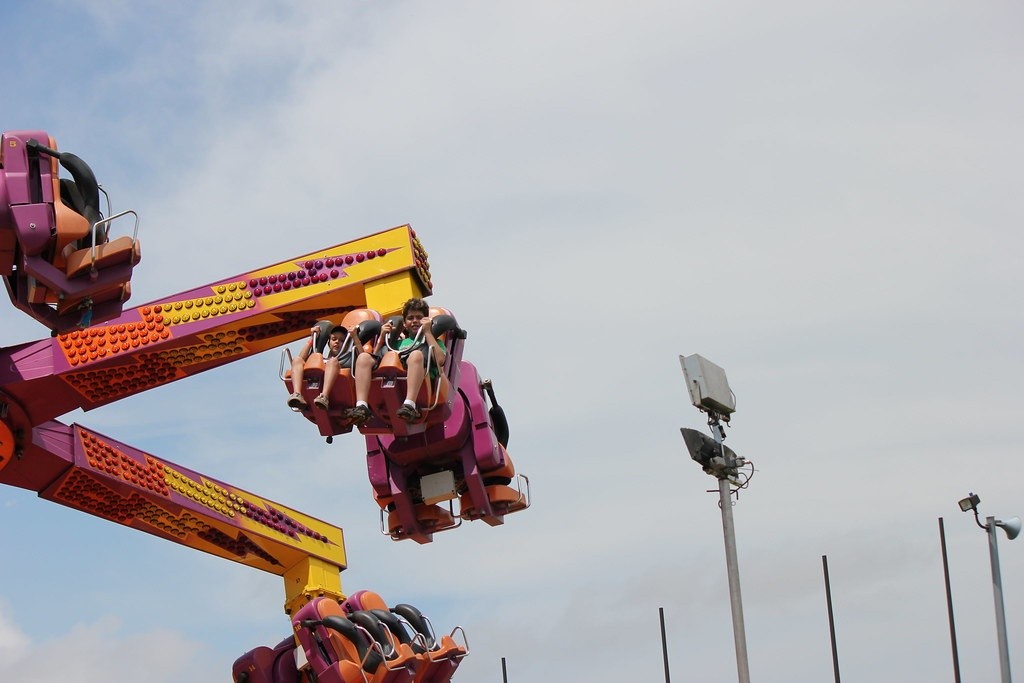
[346,298,446,424]
[287,326,365,410]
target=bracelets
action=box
[306,339,313,346]
[377,338,384,345]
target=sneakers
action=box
[395,404,422,419]
[287,392,308,411]
[313,393,329,410]
[347,404,373,422]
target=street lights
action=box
[678,354,750,683]
[958,492,1013,683]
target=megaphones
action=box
[997,517,1022,540]
